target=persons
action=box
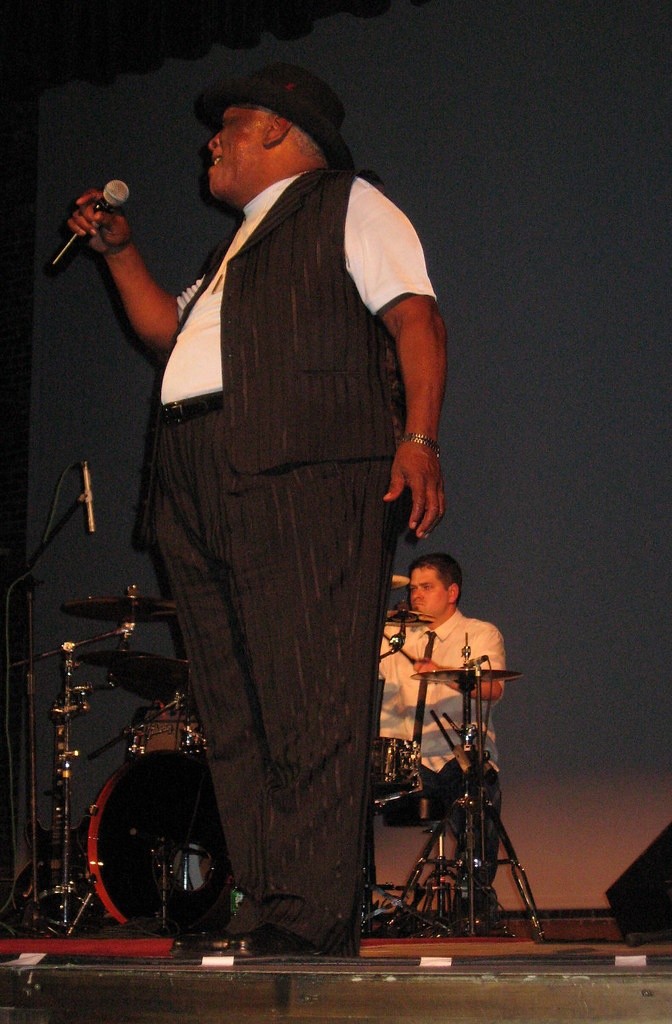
[64,59,508,964]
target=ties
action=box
[410,630,439,750]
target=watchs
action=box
[401,432,440,459]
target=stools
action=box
[383,796,462,929]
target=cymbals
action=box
[391,574,410,589]
[63,594,185,623]
[385,610,435,627]
[74,646,190,675]
[410,667,524,688]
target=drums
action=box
[84,751,236,938]
[132,716,206,772]
[371,737,417,784]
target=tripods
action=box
[375,661,548,944]
[0,603,139,939]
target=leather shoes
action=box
[169,930,249,963]
[231,930,316,960]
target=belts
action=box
[155,392,227,428]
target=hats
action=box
[192,67,357,174]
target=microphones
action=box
[463,655,489,668]
[80,459,96,533]
[48,179,130,270]
[155,835,176,858]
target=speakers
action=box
[605,822,672,946]
